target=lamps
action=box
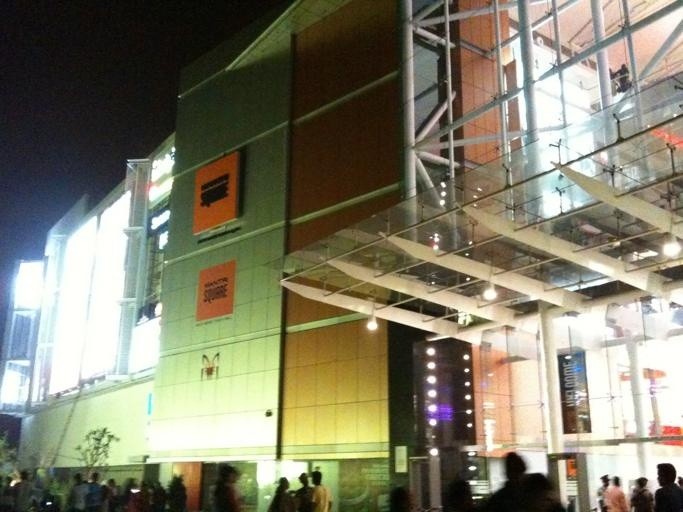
[367,304,377,330]
[483,267,497,300]
[663,217,681,257]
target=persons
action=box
[445,452,577,512]
[386,487,413,512]
[596,463,683,511]
[1,468,188,512]
[213,465,246,511]
[268,472,334,512]
